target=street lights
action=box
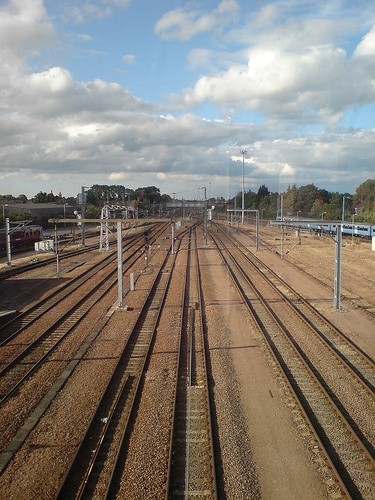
[322,212,326,221]
[296,210,301,218]
[342,195,351,221]
[3,201,9,228]
[64,202,69,230]
[201,187,207,200]
[239,149,248,226]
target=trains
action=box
[275,217,375,240]
[0,224,43,252]
